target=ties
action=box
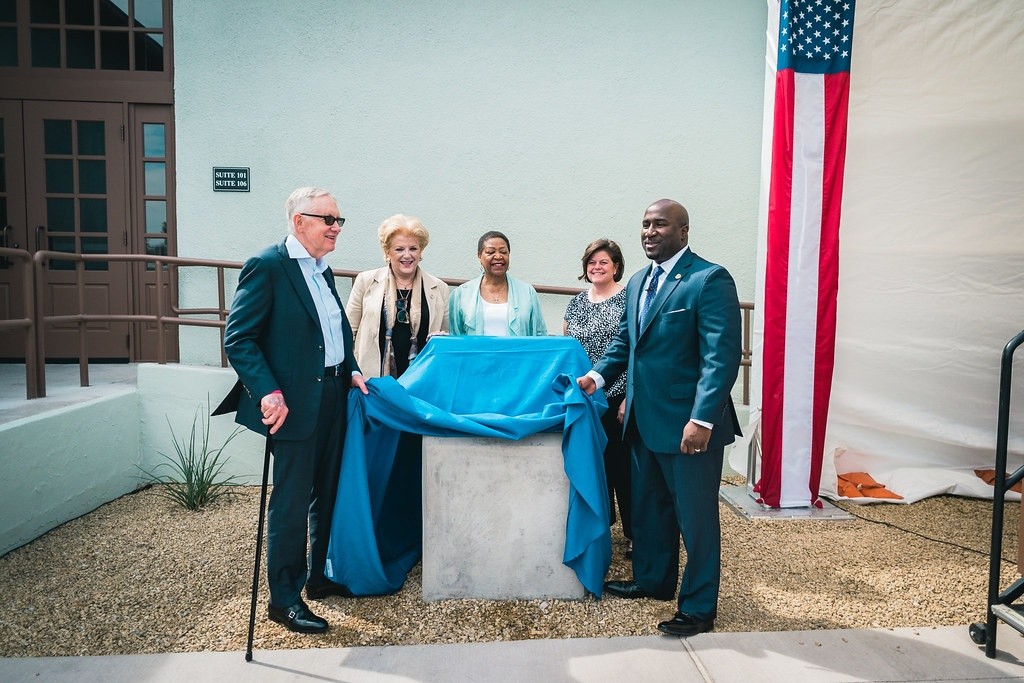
[640,265,665,337]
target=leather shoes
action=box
[605,580,675,601]
[268,603,329,633]
[307,583,352,600]
[658,610,715,636]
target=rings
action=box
[694,448,700,453]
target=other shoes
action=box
[625,540,632,559]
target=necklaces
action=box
[482,278,507,303]
[393,274,414,300]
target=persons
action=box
[210,183,369,633]
[344,214,450,381]
[562,238,635,559]
[576,198,744,636]
[448,231,548,338]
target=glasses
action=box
[395,299,410,324]
[300,213,346,227]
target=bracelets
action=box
[266,389,283,394]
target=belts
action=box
[323,362,345,377]
